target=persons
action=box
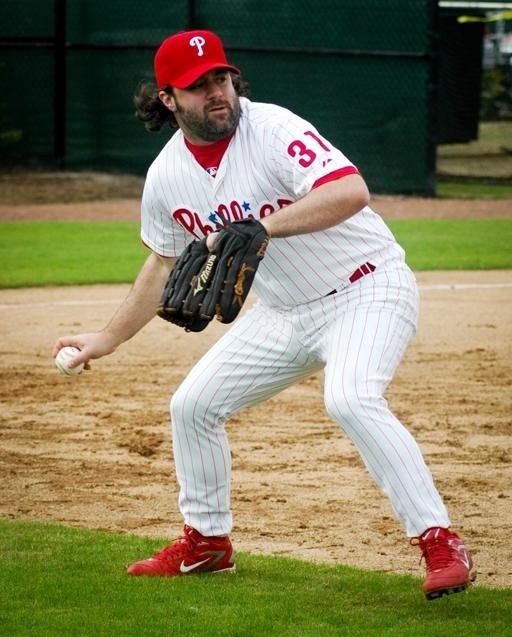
[50,27,478,601]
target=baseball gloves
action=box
[155,218,269,332]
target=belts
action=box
[328,263,376,291]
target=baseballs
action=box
[55,346,85,375]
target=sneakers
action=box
[126,524,237,577]
[417,527,476,601]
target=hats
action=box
[154,28,241,90]
